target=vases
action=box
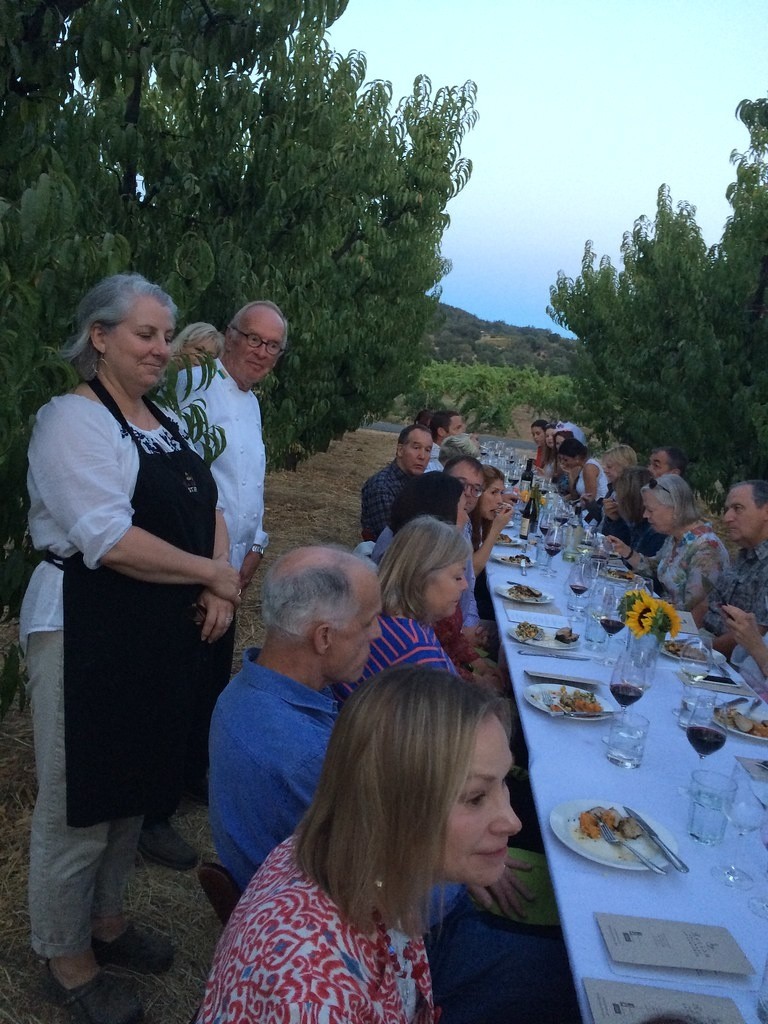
[623,624,667,668]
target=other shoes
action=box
[138,817,200,870]
[187,772,211,805]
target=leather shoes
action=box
[90,926,174,973]
[42,957,146,1024]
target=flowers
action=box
[617,590,682,641]
[520,491,546,505]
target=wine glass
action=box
[747,803,768,919]
[709,761,768,891]
[678,695,728,803]
[672,635,714,718]
[481,440,654,669]
[601,645,646,749]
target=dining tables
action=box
[477,443,768,1024]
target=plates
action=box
[494,537,522,546]
[549,799,678,871]
[503,520,514,528]
[507,627,582,650]
[598,567,646,583]
[710,710,768,741]
[660,640,726,665]
[494,586,554,604]
[490,553,537,567]
[522,684,613,721]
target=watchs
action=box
[249,546,264,560]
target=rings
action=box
[237,588,242,596]
[226,617,233,621]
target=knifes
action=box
[517,651,591,661]
[623,806,689,873]
[520,558,527,576]
[548,709,627,717]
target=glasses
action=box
[649,478,671,495]
[458,478,483,498]
[233,327,287,355]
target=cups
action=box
[606,712,650,770]
[687,769,738,846]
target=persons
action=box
[167,322,225,369]
[330,408,768,856]
[208,543,584,1024]
[197,666,521,1024]
[137,299,290,867]
[19,272,242,1024]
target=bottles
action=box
[521,459,534,493]
[562,502,583,562]
[519,486,537,539]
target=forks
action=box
[541,690,574,717]
[598,822,667,875]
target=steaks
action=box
[734,712,754,733]
[529,587,542,597]
[555,627,580,644]
[587,806,643,839]
